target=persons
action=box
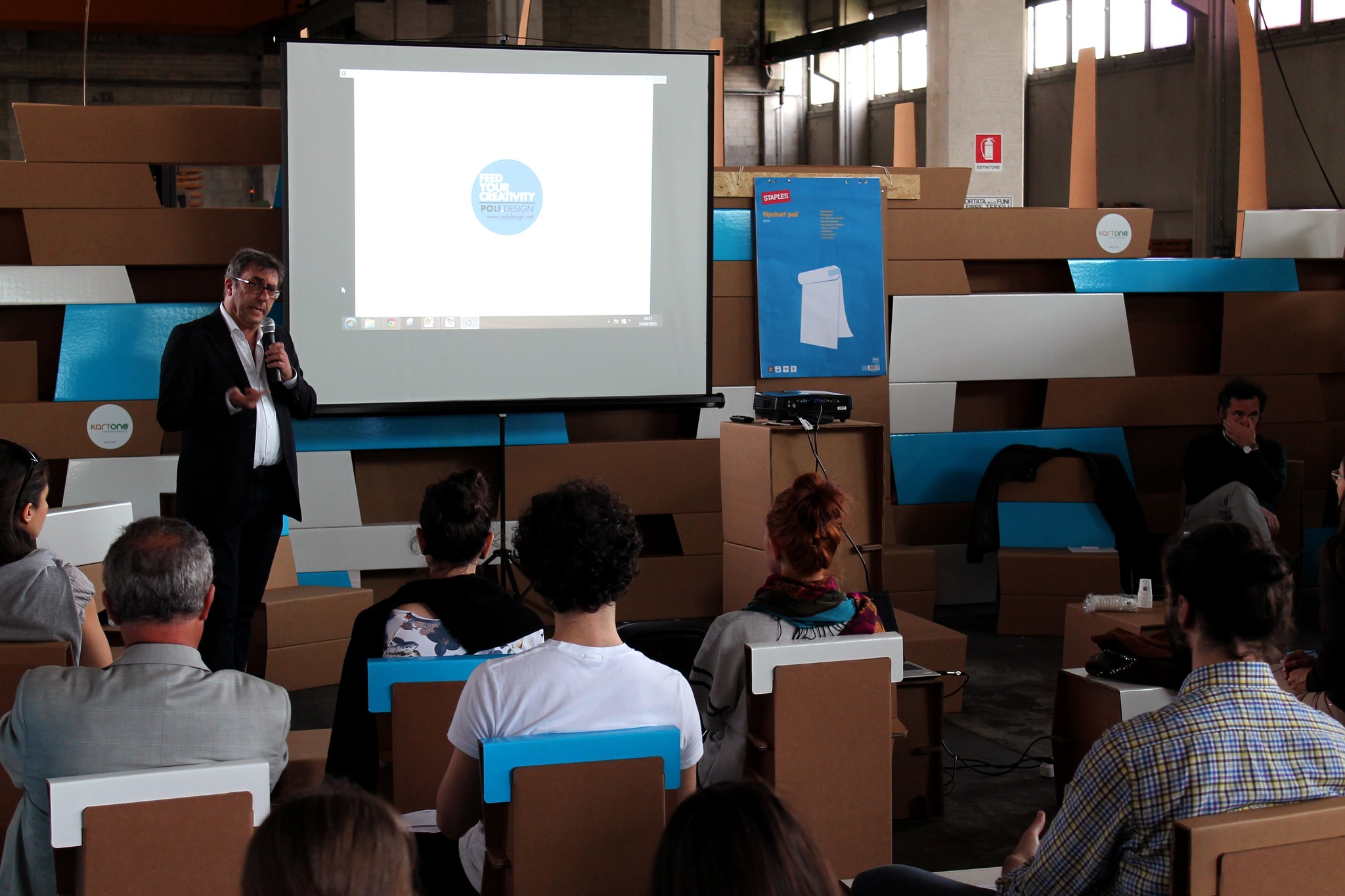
[682,471,890,788]
[844,523,1345,896]
[0,440,114,671]
[405,476,704,896]
[1159,374,1345,732]
[651,781,846,896]
[157,247,317,672]
[317,465,546,805]
[238,781,416,896]
[0,516,292,896]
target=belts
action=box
[252,469,274,480]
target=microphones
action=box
[259,317,282,382]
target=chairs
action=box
[476,721,685,896]
[1174,794,1345,896]
[159,488,374,695]
[0,640,76,856]
[34,496,138,663]
[992,450,1122,639]
[43,752,275,896]
[368,646,535,810]
[734,626,906,869]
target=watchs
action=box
[1241,443,1258,453]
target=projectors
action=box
[753,390,853,424]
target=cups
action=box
[1090,595,1137,611]
[1137,579,1152,608]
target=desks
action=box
[1060,603,1164,668]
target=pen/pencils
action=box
[1273,650,1319,673]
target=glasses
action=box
[235,277,282,300]
[13,444,39,513]
[1330,469,1345,481]
